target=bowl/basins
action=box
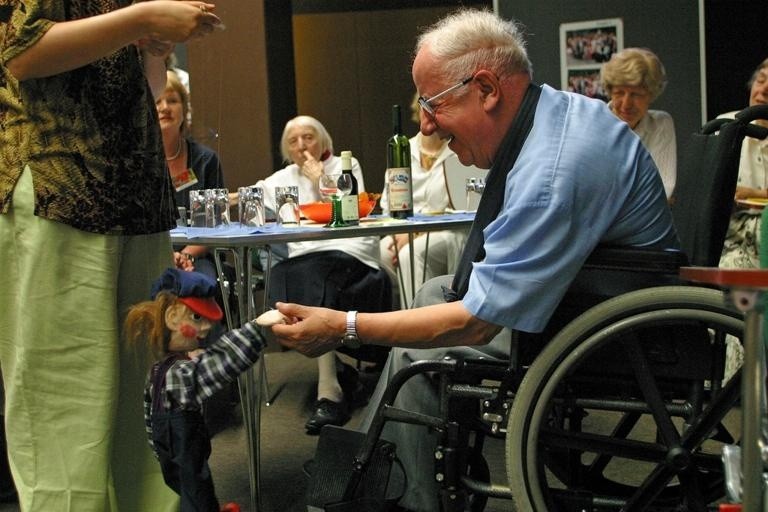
[298,201,376,224]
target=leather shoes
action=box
[304,392,349,430]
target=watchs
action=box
[341,309,362,350]
[182,253,195,263]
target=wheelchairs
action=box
[298,101,767,512]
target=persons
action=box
[152,69,223,220]
[599,47,678,202]
[223,115,366,220]
[0,0,225,512]
[710,57,768,270]
[379,91,457,310]
[272,7,701,512]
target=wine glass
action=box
[318,173,353,228]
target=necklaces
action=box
[166,136,182,161]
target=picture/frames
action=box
[558,17,624,103]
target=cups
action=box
[237,186,265,228]
[275,186,300,227]
[188,188,230,231]
[719,442,767,504]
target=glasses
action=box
[418,75,500,120]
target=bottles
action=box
[386,105,415,220]
[337,150,360,226]
